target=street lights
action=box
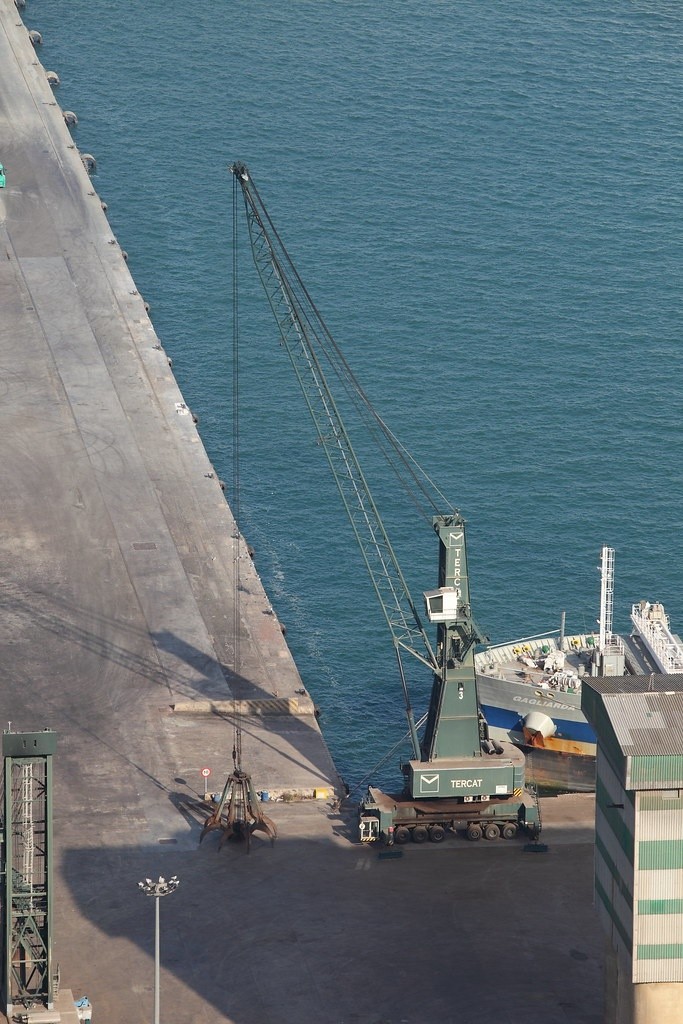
[137,875,180,1024]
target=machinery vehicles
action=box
[200,157,546,855]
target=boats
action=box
[474,547,683,789]
